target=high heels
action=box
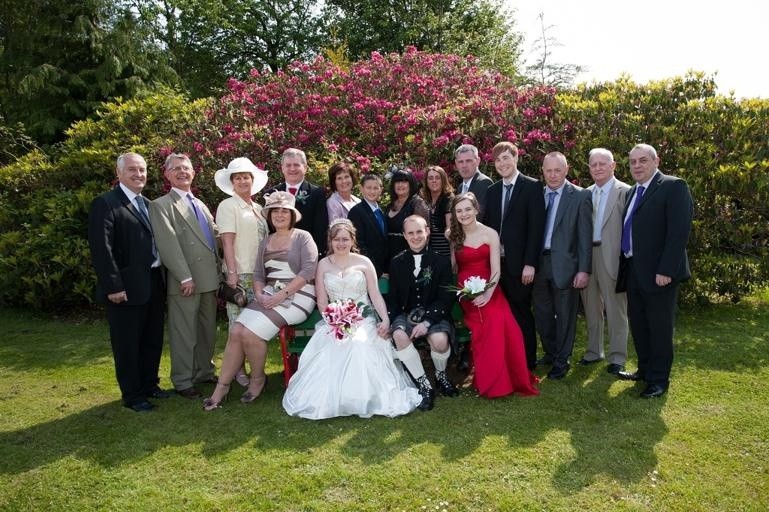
[203,375,267,411]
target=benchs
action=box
[279,272,471,389]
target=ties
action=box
[543,192,558,242]
[464,185,468,192]
[374,209,384,232]
[500,184,512,245]
[592,188,602,223]
[289,188,297,195]
[187,193,213,249]
[622,186,645,254]
[135,196,157,258]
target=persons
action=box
[384,213,459,412]
[531,150,593,381]
[485,140,546,371]
[201,189,319,413]
[446,191,542,401]
[578,146,629,376]
[278,216,424,421]
[148,151,223,402]
[613,143,693,400]
[213,142,495,391]
[86,150,170,413]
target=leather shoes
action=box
[415,374,433,410]
[434,370,459,397]
[535,353,666,398]
[131,387,201,411]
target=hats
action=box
[261,191,302,223]
[214,157,268,196]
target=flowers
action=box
[320,296,374,341]
[439,272,500,324]
[297,190,311,205]
[422,266,433,286]
